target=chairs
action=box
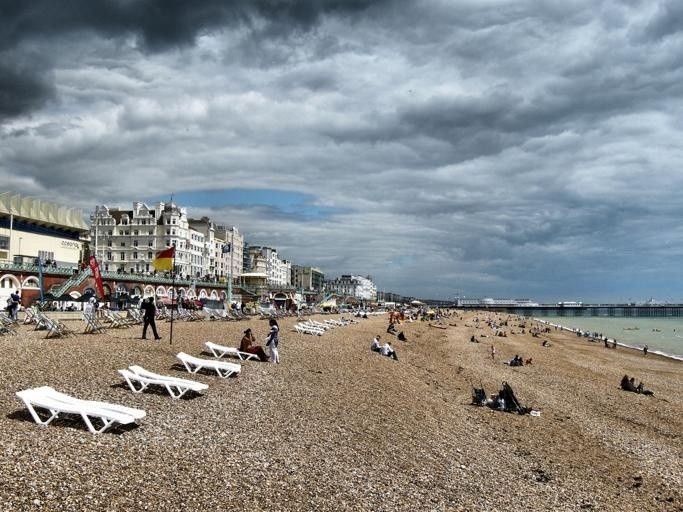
[293,317,360,336]
[205,340,261,361]
[16,385,146,434]
[118,365,209,400]
[0,306,312,339]
[177,351,241,378]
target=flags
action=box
[152,247,174,273]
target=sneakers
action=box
[261,356,279,364]
[142,336,162,339]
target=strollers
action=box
[469,377,486,406]
[498,380,525,415]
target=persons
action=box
[620,374,655,395]
[341,312,369,322]
[177,294,203,314]
[240,319,280,364]
[498,380,532,416]
[231,300,256,313]
[138,296,165,340]
[643,343,649,355]
[8,290,21,319]
[371,307,616,366]
[33,301,77,311]
